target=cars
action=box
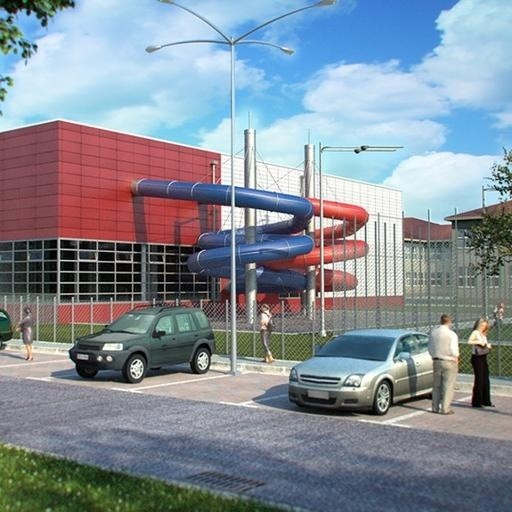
[287,327,433,415]
[1,308,13,349]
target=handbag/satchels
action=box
[476,344,489,356]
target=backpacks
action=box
[264,312,276,332]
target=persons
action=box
[16,308,35,362]
[468,318,497,410]
[258,303,275,364]
[426,314,460,415]
[495,298,505,326]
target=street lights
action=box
[145,1,336,376]
[318,139,404,338]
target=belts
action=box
[433,358,443,360]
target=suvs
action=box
[70,304,216,383]
